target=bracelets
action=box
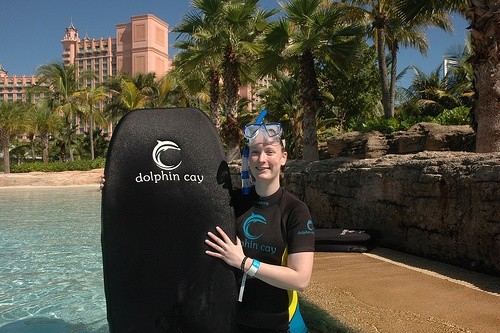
[245,258,260,277]
[240,256,248,272]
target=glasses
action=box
[243,123,284,142]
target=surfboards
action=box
[100,107,243,333]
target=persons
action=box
[99,122,316,333]
